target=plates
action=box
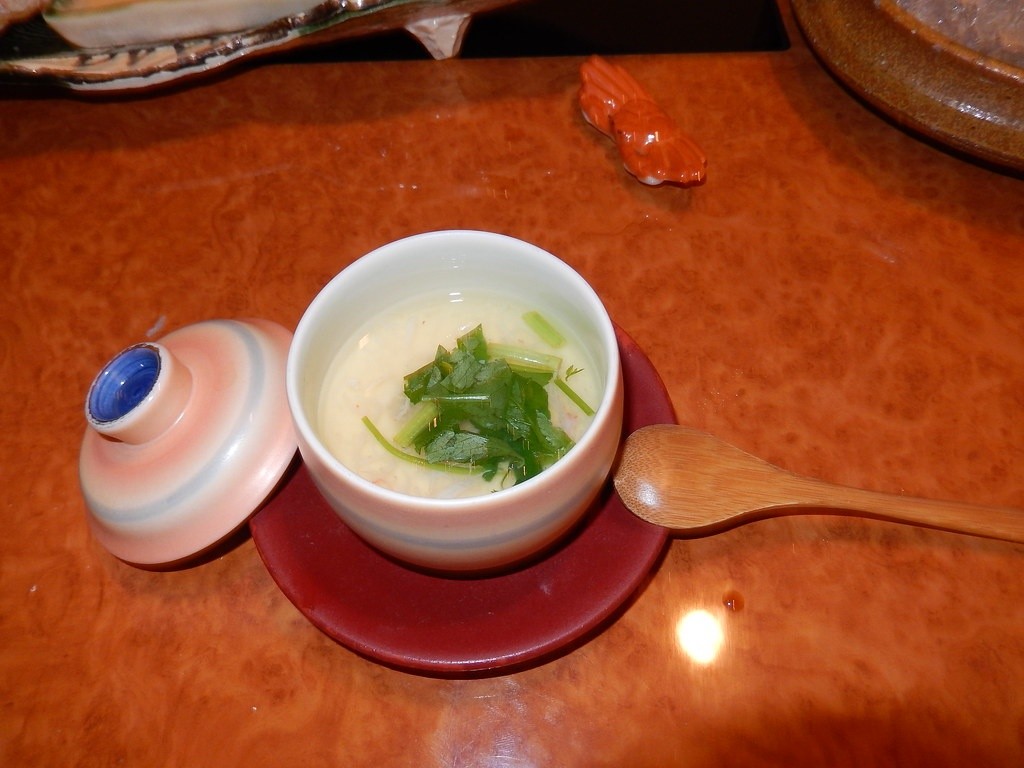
[42,0,323,49]
[249,298,681,676]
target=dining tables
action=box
[1,48,1023,768]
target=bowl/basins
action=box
[791,0,1023,172]
[283,229,626,572]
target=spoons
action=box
[613,422,1024,561]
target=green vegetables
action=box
[359,311,599,495]
